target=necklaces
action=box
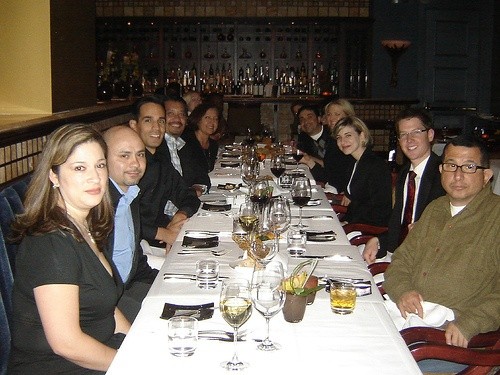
[65,210,95,244]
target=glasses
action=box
[440,162,487,174]
[395,129,429,142]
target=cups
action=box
[241,146,260,185]
[248,219,279,268]
[287,230,306,255]
[195,260,220,291]
[267,198,291,234]
[303,275,318,305]
[231,216,254,250]
[169,316,199,357]
[330,282,356,315]
[283,291,307,323]
[249,177,269,206]
[239,203,260,232]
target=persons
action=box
[182,103,220,173]
[129,96,201,271]
[332,114,392,227]
[160,93,211,198]
[290,102,331,178]
[298,99,356,192]
[6,123,132,375]
[104,126,160,325]
[357,110,447,289]
[382,134,500,373]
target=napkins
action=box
[385,299,456,333]
[323,181,339,194]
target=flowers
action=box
[282,272,328,296]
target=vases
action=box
[282,294,308,322]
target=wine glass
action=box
[251,260,287,351]
[291,177,312,229]
[219,278,254,370]
[292,144,305,171]
[270,155,285,188]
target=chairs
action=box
[324,190,500,375]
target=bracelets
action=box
[375,237,380,249]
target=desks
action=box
[102,143,423,375]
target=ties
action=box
[400,171,417,241]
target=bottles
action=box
[169,45,322,59]
[237,63,273,98]
[168,63,197,94]
[311,62,338,96]
[201,63,235,95]
[274,62,309,95]
[97,56,160,101]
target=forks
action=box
[199,330,248,339]
[177,248,232,256]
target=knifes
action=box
[290,255,351,259]
